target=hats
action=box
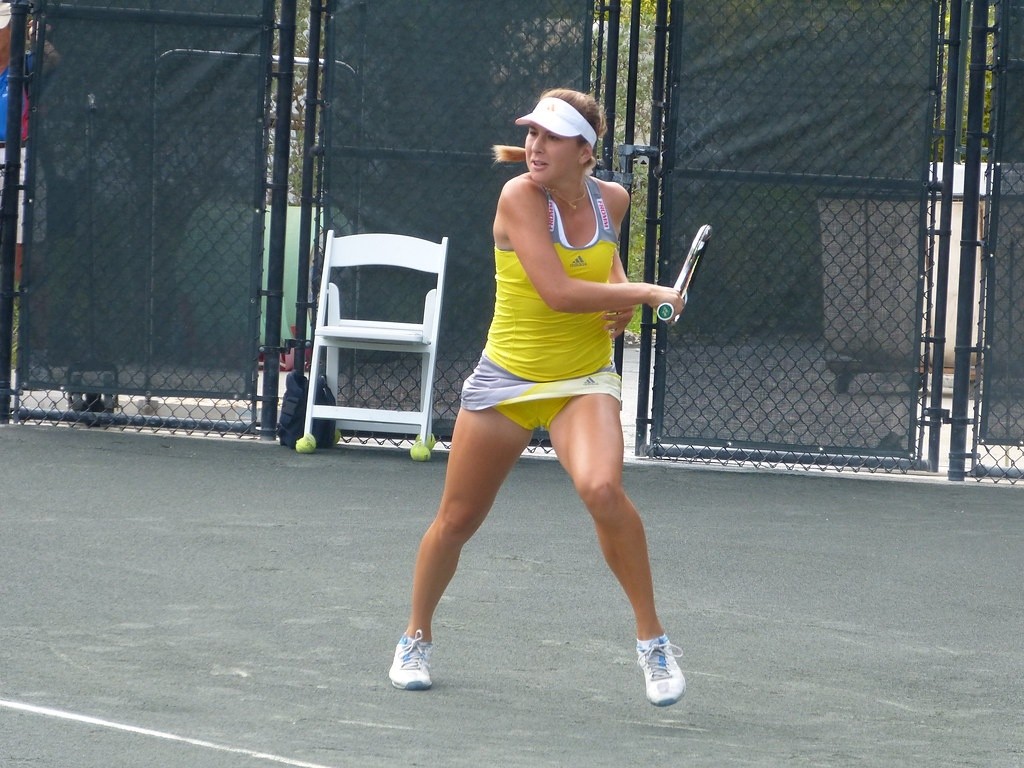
[515,97,598,148]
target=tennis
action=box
[415,433,436,451]
[410,441,431,461]
[334,428,340,444]
[295,433,316,453]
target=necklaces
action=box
[544,185,586,208]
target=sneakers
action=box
[637,639,687,706]
[388,628,434,690]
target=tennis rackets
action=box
[655,223,713,325]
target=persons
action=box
[388,87,687,706]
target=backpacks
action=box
[275,369,336,450]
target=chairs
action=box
[295,229,450,461]
